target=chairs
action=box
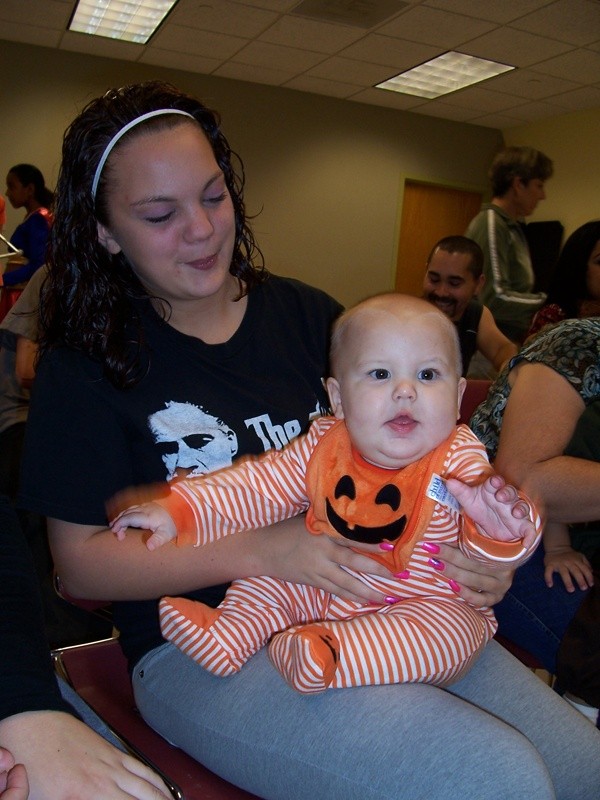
[8,422,263,800]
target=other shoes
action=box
[521,650,558,689]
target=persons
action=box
[464,148,557,373]
[110,293,543,694]
[468,318,599,721]
[528,220,599,331]
[0,164,177,800]
[421,236,520,372]
[10,81,600,800]
